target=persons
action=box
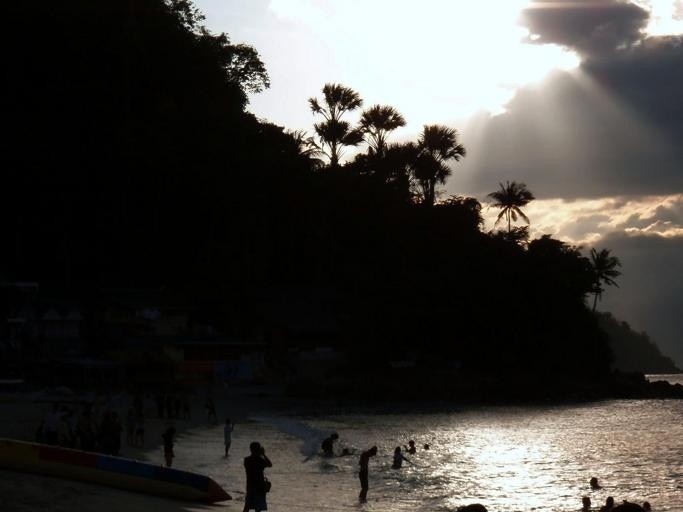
[243,441,273,512]
[391,445,408,468]
[314,431,339,458]
[222,419,235,458]
[404,440,418,455]
[357,446,378,500]
[589,477,602,490]
[598,497,614,511]
[576,498,592,512]
[642,501,651,510]
[338,447,354,456]
[422,443,429,449]
[162,426,174,468]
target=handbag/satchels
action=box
[265,477,271,492]
